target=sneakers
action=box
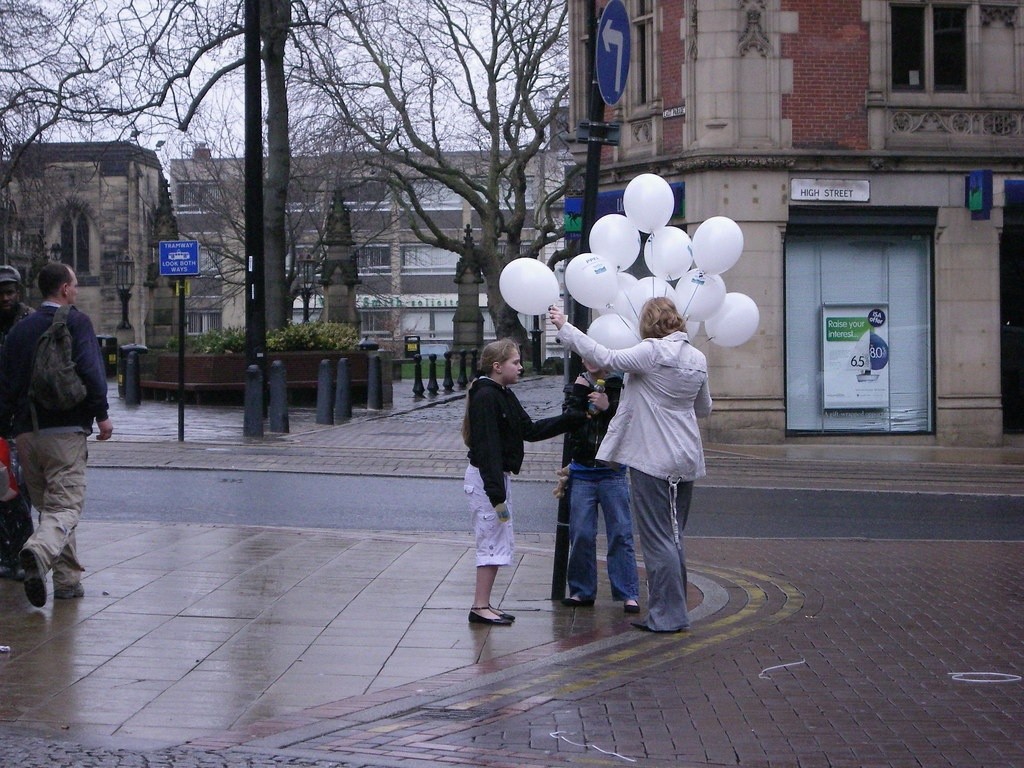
[623,600,640,613]
[561,596,594,607]
[19,547,48,609]
[54,583,86,599]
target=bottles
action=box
[496,502,510,522]
[589,379,605,415]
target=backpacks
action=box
[26,303,86,438]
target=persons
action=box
[460,339,591,624]
[0,262,113,607]
[562,368,641,615]
[549,297,711,632]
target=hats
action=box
[0,265,21,282]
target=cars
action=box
[420,344,451,360]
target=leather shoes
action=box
[468,604,515,625]
[630,621,682,634]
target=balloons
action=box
[499,174,760,349]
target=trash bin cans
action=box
[117,344,149,397]
[404,335,421,358]
[95,334,117,377]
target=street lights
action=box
[298,254,321,324]
[113,249,138,400]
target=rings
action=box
[550,314,554,319]
